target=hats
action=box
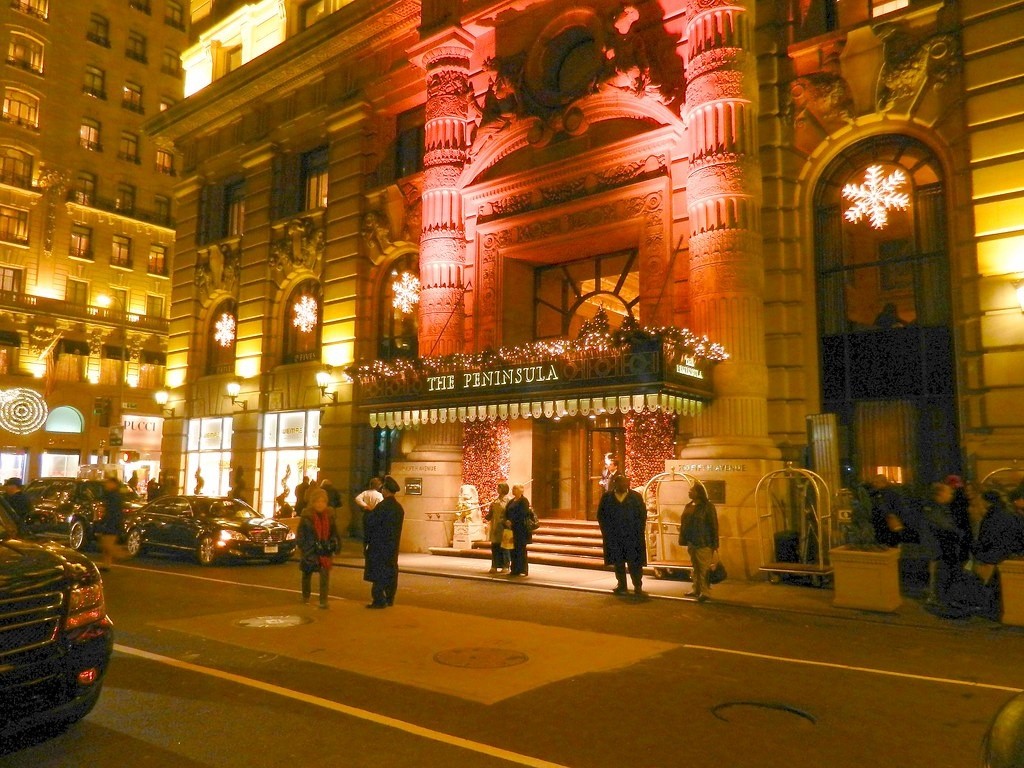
[383,475,400,493]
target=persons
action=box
[924,474,1024,607]
[97,478,132,573]
[606,458,624,491]
[319,479,341,508]
[678,480,720,604]
[504,482,533,577]
[5,477,33,525]
[294,476,310,517]
[354,478,385,555]
[870,474,894,544]
[596,474,648,596]
[128,470,138,490]
[297,489,342,609]
[147,477,157,502]
[488,482,512,573]
[303,479,318,507]
[362,475,405,609]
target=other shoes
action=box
[501,567,510,572]
[634,585,642,593]
[613,586,627,593]
[697,595,710,601]
[685,591,701,597]
[489,568,497,573]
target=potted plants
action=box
[995,515,1024,626]
[830,465,903,615]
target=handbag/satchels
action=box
[706,549,728,584]
[313,540,335,557]
[519,497,540,532]
[500,525,515,550]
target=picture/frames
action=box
[404,477,422,494]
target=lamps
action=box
[228,380,248,411]
[154,386,175,418]
[316,369,339,404]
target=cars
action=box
[0,477,121,550]
[124,496,298,567]
[1,486,116,753]
